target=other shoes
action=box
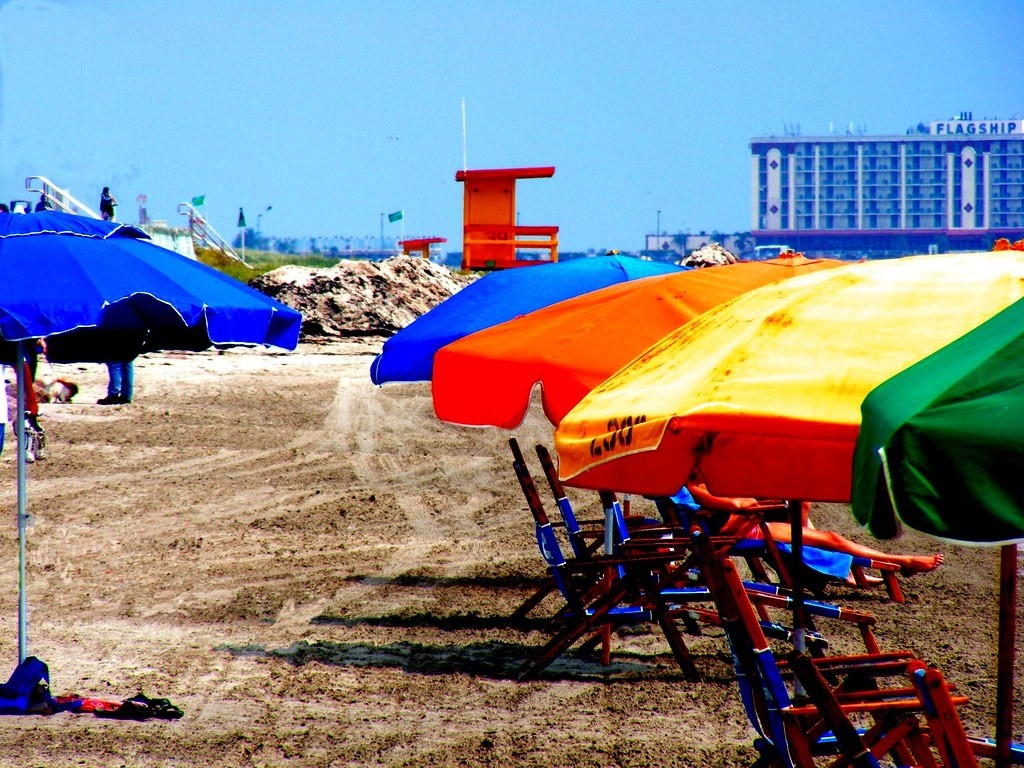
[119,396,131,405]
[96,394,119,404]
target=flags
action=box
[388,210,403,223]
[192,195,205,206]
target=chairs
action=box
[506,437,1024,768]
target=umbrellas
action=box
[366,240,1024,768]
[0,211,302,668]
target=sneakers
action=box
[32,417,48,461]
[11,411,36,463]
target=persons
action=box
[5,382,45,462]
[0,195,53,214]
[97,362,133,404]
[100,186,117,221]
[686,470,944,576]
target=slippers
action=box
[123,692,184,719]
[93,701,152,721]
